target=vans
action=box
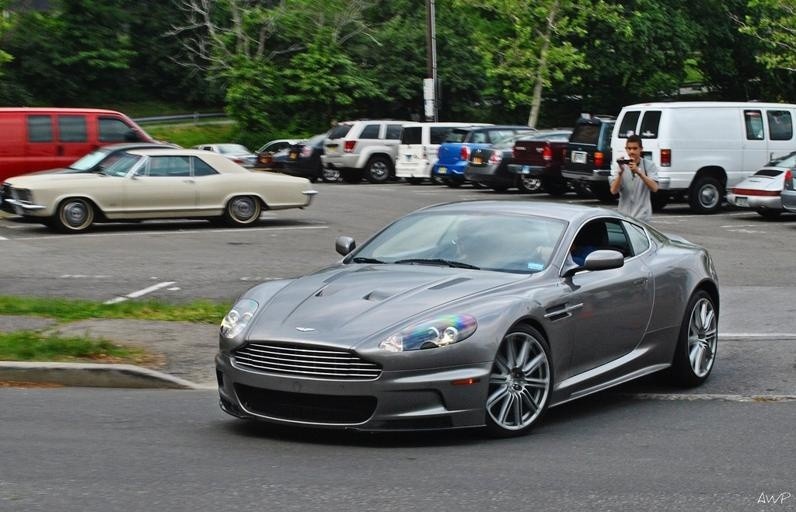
[0,104,178,189]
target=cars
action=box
[6,138,318,230]
[182,130,325,185]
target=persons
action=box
[309,136,328,182]
[608,135,661,223]
[531,219,586,272]
[329,120,338,128]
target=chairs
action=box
[571,225,601,248]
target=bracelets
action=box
[618,171,622,176]
[637,168,641,176]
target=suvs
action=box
[325,101,794,231]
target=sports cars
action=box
[211,196,719,438]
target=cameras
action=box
[617,159,633,165]
